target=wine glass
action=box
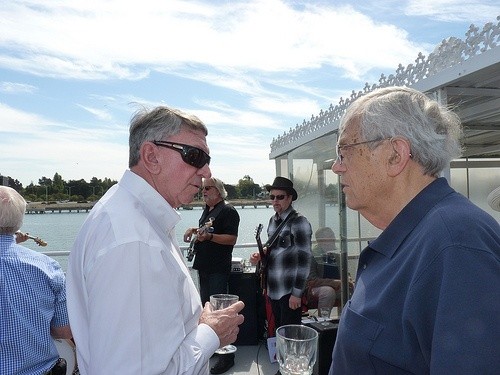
[210,294,239,354]
[321,308,329,326]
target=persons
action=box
[328,85,500,375]
[306,227,355,317]
[66,100,245,375]
[184,176,240,375]
[250,177,312,375]
[0,185,73,375]
[15,231,29,244]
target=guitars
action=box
[254,223,269,289]
[185,217,216,262]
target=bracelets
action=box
[208,233,214,241]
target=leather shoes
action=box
[210,357,234,374]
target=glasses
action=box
[336,137,413,165]
[151,138,210,168]
[202,186,218,191]
[267,194,289,201]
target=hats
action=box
[266,176,298,201]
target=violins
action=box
[14,230,48,247]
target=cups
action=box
[245,260,250,271]
[276,325,319,375]
